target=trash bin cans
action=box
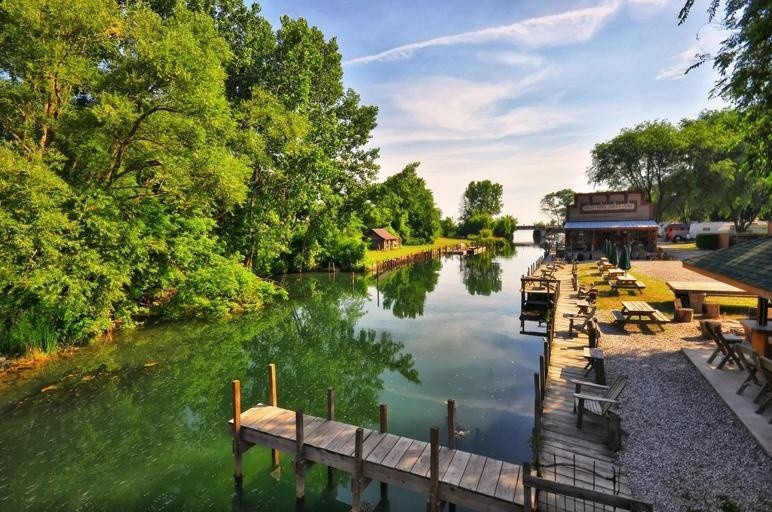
[719,233,730,249]
[638,247,647,259]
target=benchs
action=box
[649,309,670,331]
[607,281,647,296]
[610,311,626,334]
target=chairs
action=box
[529,261,564,287]
[570,373,628,439]
[566,282,600,336]
[701,321,772,426]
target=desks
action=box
[597,257,625,283]
[610,275,643,295]
[613,301,665,332]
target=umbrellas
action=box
[603,238,608,257]
[618,244,632,278]
[606,240,612,261]
[609,242,618,268]
